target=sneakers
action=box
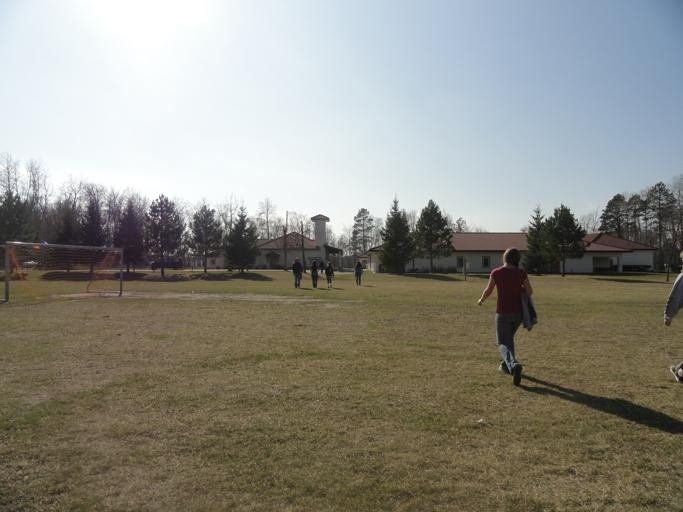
[670,365,683,383]
[498,362,523,386]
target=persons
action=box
[324,262,334,288]
[351,261,362,286]
[292,257,301,288]
[309,260,317,288]
[475,247,533,386]
[662,272,683,382]
